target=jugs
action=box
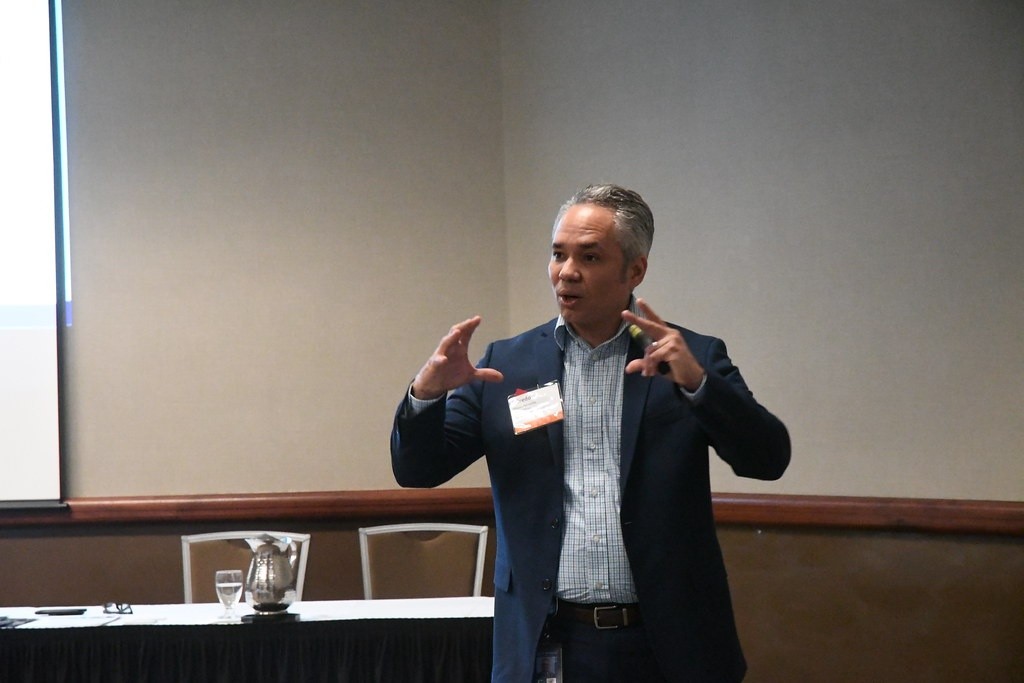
[246,534,297,614]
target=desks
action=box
[1,597,495,683]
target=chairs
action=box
[180,531,310,605]
[359,523,488,600]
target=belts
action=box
[552,601,650,630]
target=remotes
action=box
[628,325,670,375]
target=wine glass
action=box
[215,569,243,622]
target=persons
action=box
[390,180,793,682]
